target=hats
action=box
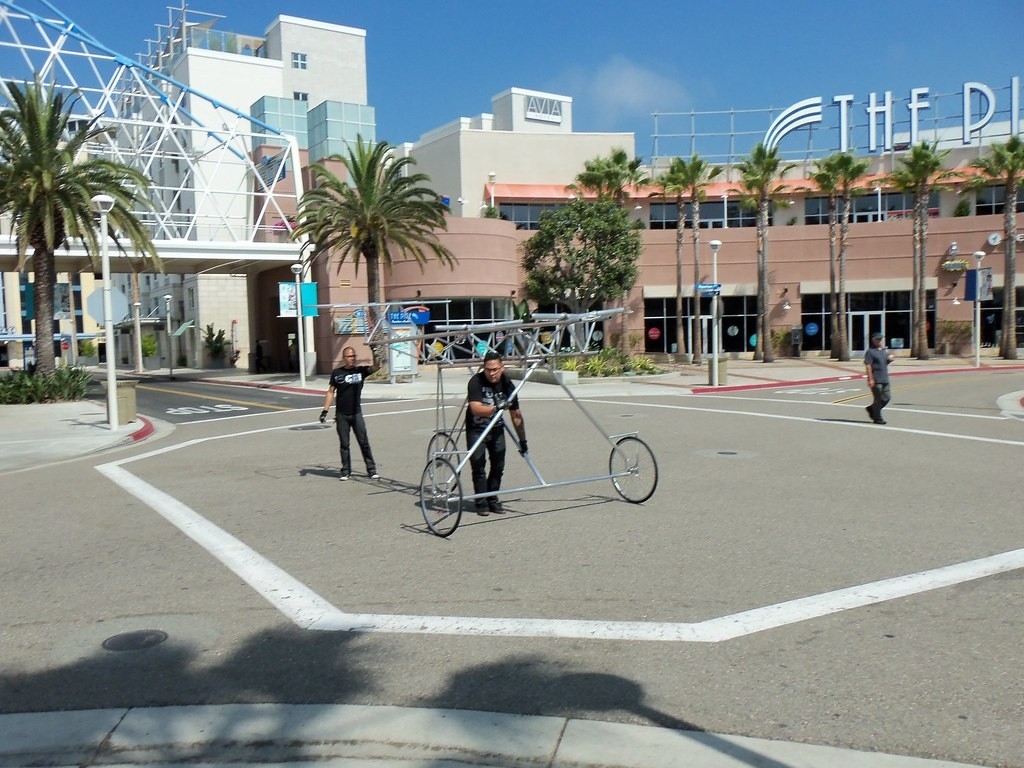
[872,332,883,338]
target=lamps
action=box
[477,200,489,209]
[953,280,957,288]
[788,197,795,206]
[952,297,961,306]
[417,291,421,296]
[956,185,963,195]
[784,287,788,292]
[635,200,642,209]
[782,301,791,309]
[511,290,516,296]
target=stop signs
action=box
[62,342,69,351]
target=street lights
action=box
[973,250,986,367]
[291,264,305,388]
[163,294,172,377]
[134,302,143,373]
[708,238,722,386]
[91,194,120,431]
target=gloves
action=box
[518,440,528,457]
[493,399,513,413]
[369,340,378,352]
[320,410,328,423]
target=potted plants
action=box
[142,330,161,370]
[79,338,100,366]
[771,329,788,358]
[936,322,959,354]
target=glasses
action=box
[343,354,357,358]
[484,365,503,373]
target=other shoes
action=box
[474,502,490,515]
[339,475,348,480]
[874,419,886,424]
[866,406,875,420]
[370,473,380,478]
[488,500,506,514]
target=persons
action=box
[255,340,267,374]
[319,343,380,481]
[864,333,895,424]
[289,339,298,372]
[466,352,528,516]
